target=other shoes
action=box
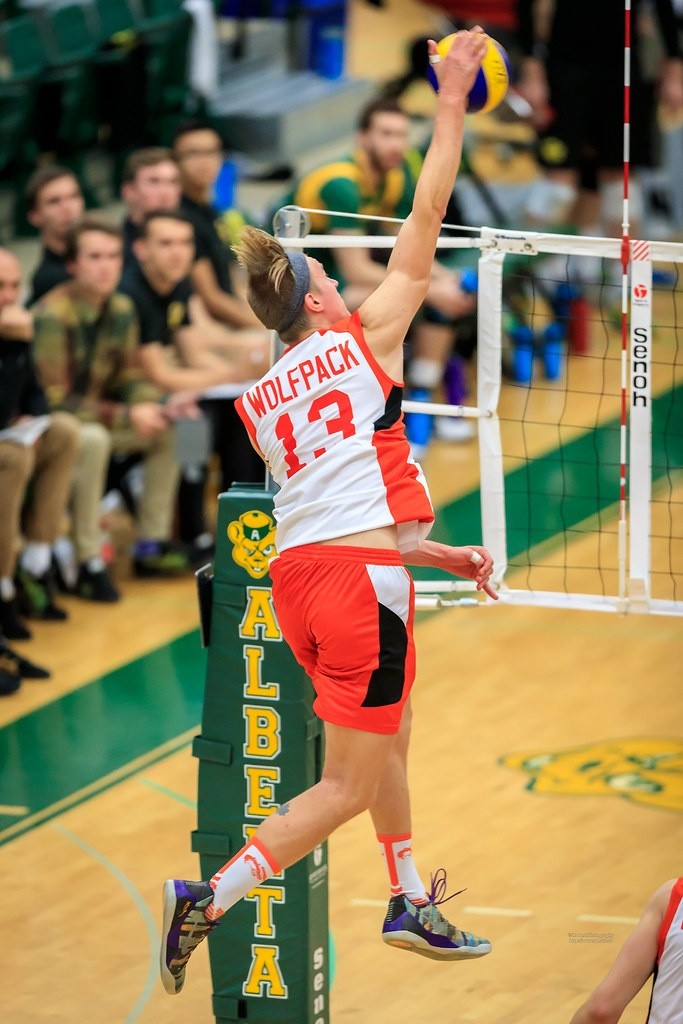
[131,544,190,576]
[1,537,74,695]
[77,562,120,602]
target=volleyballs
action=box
[426,31,512,114]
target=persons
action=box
[174,111,286,327]
[118,210,274,561]
[564,864,683,1024]
[289,97,496,437]
[0,250,84,633]
[19,159,84,306]
[157,33,507,997]
[501,0,683,285]
[108,147,280,358]
[20,211,194,605]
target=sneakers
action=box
[383,895,491,961]
[160,880,221,995]
